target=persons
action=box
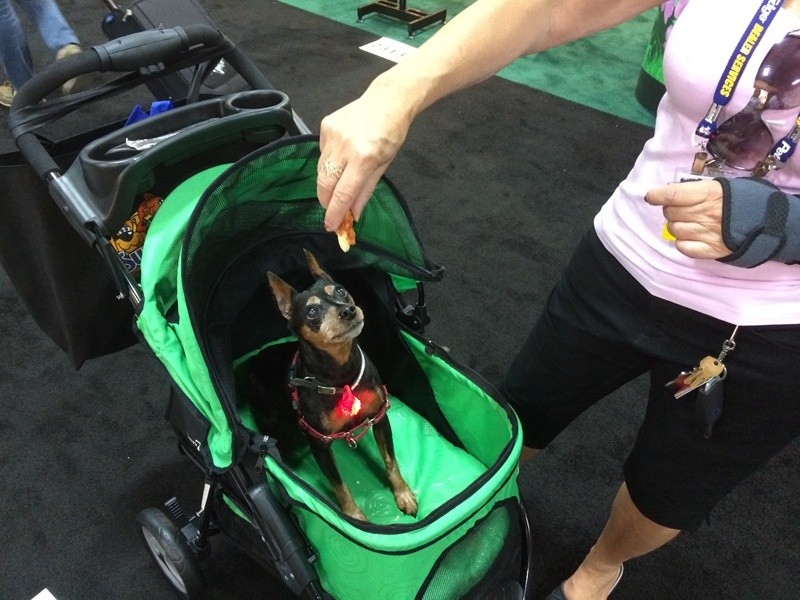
[0,0,83,109]
[316,0,799,600]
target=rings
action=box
[320,158,343,180]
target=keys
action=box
[662,324,743,439]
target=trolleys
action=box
[9,23,534,599]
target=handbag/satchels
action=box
[1,33,270,371]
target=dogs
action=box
[265,247,420,525]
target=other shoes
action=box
[545,562,625,600]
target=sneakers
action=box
[56,43,92,96]
[0,81,47,108]
